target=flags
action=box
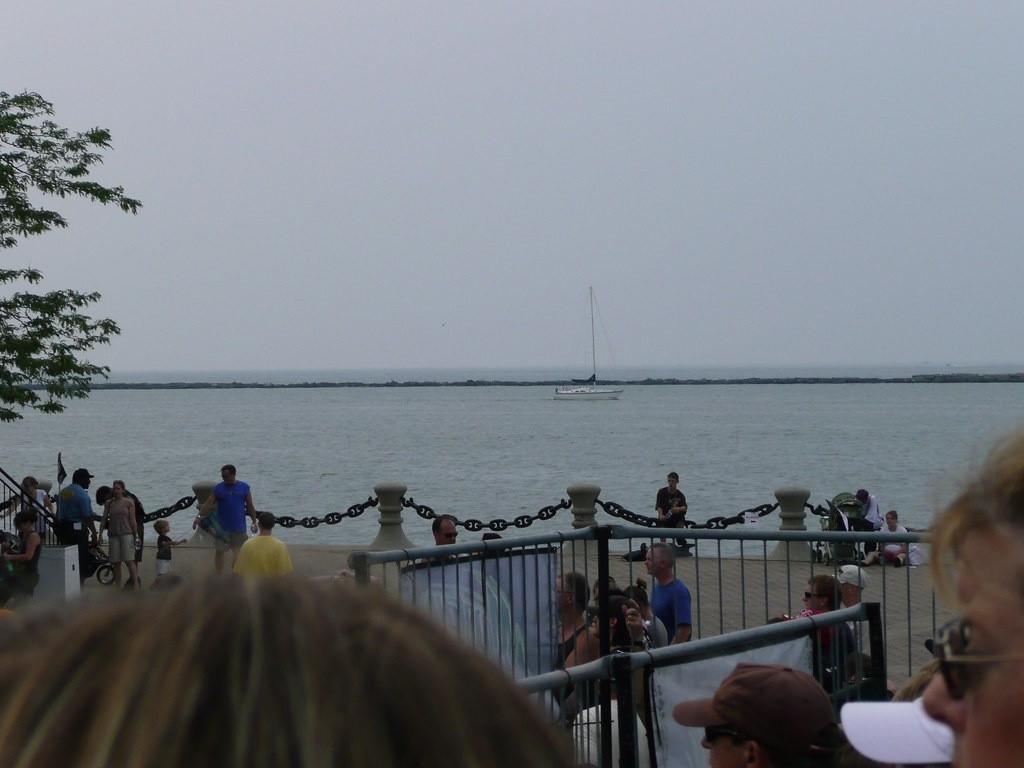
[57,452,68,486]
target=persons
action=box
[193,465,259,572]
[922,431,1024,768]
[234,511,293,597]
[854,489,885,558]
[794,566,870,724]
[700,662,837,768]
[855,509,908,568]
[543,542,692,768]
[50,468,98,594]
[657,472,694,556]
[96,480,145,593]
[0,476,56,547]
[431,516,457,545]
[0,510,42,597]
[153,520,188,577]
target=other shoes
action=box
[893,556,903,567]
[676,538,687,545]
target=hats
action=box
[840,695,955,764]
[588,595,632,615]
[673,662,841,753]
[855,489,868,498]
[832,565,869,588]
[73,468,95,479]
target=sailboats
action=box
[552,287,626,400]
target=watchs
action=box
[197,514,201,518]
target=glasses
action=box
[936,617,1024,700]
[805,592,820,598]
[705,726,738,742]
[441,531,458,538]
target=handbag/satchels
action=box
[104,520,109,529]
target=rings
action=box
[194,521,196,524]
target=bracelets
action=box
[90,532,98,537]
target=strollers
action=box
[45,512,117,586]
[810,492,865,566]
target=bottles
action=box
[5,558,16,577]
